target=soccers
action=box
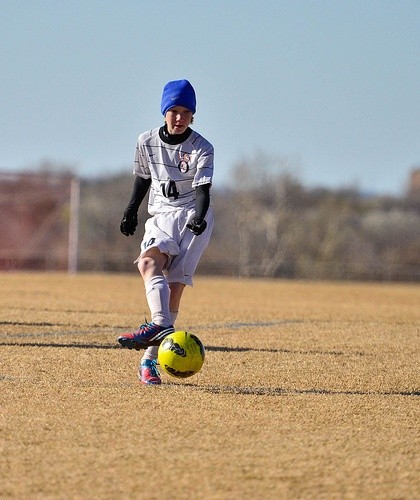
[158,331,205,378]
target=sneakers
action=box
[137,359,161,385]
[118,317,175,351]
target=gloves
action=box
[186,217,207,236]
[120,211,138,236]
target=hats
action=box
[161,79,196,117]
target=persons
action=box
[120,80,215,386]
